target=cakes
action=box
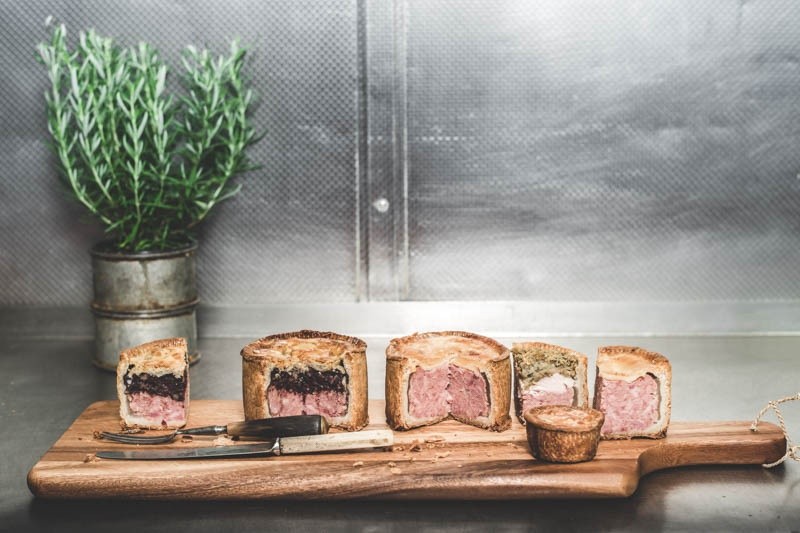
[517,402,605,463]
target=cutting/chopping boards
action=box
[27,398,785,501]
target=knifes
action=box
[96,429,394,460]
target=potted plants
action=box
[37,12,273,373]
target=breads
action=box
[116,335,193,436]
[509,340,591,430]
[237,329,368,431]
[590,344,674,444]
[382,332,514,434]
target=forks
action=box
[98,412,334,444]
